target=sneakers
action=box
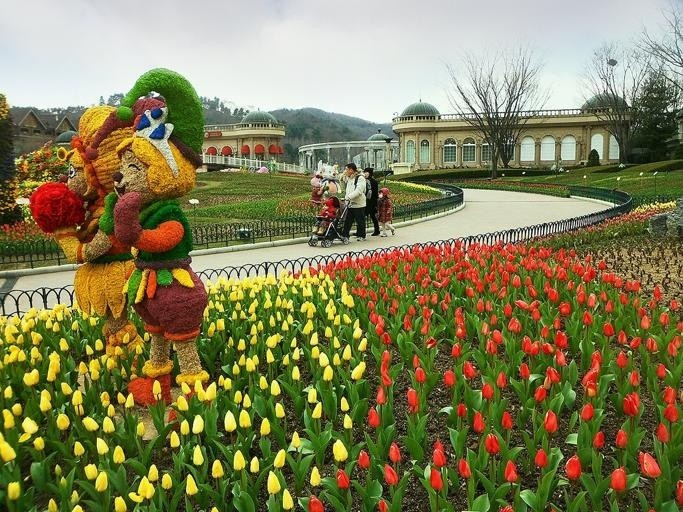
[370,231,381,235]
[381,233,387,237]
[353,232,367,241]
[391,228,396,235]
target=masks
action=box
[378,194,383,198]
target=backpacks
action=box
[354,175,372,199]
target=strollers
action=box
[307,198,349,248]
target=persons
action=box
[311,162,396,241]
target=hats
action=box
[362,167,373,174]
[380,188,389,195]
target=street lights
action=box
[384,136,392,171]
